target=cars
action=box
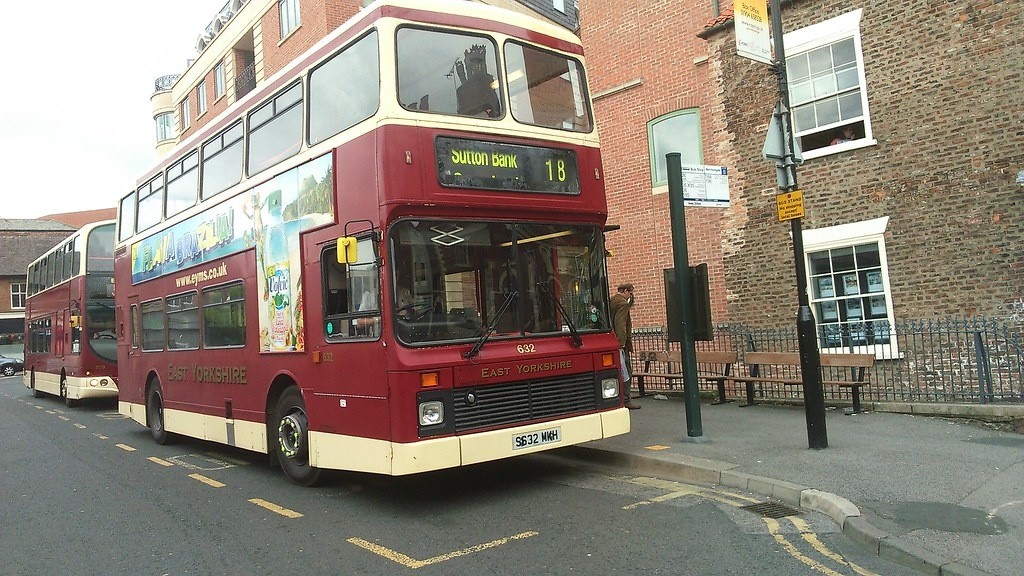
[0,355,24,377]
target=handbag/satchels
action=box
[620,349,630,382]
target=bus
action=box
[23,218,118,408]
[113,2,631,488]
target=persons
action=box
[94,318,116,338]
[610,282,641,409]
[357,259,443,325]
[831,126,855,145]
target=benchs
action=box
[728,351,875,415]
[631,350,738,407]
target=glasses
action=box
[628,292,633,295]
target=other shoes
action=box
[625,403,641,410]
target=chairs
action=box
[347,276,372,311]
